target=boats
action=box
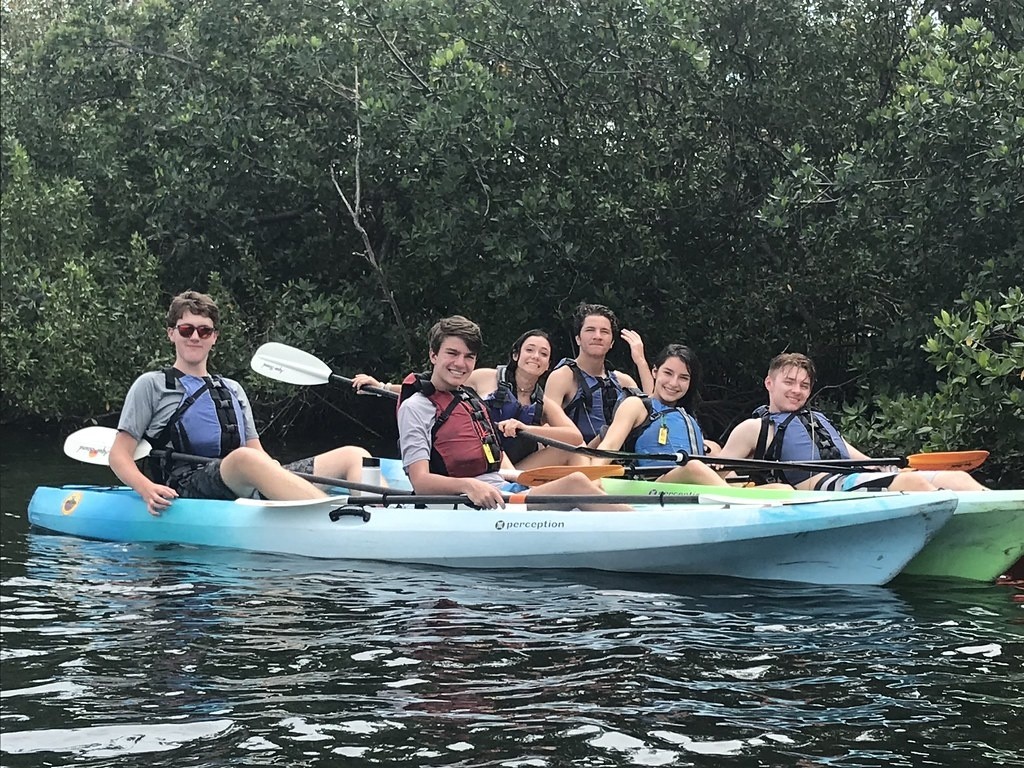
[25,474,960,587]
[591,476,1024,584]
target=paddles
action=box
[514,448,993,488]
[61,425,412,498]
[249,341,604,460]
[574,444,878,474]
[346,493,769,507]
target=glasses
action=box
[174,324,216,340]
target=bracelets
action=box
[377,381,392,397]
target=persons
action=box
[396,314,635,511]
[542,303,654,454]
[708,352,992,491]
[351,328,591,485]
[592,343,744,487]
[107,291,391,516]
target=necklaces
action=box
[517,382,534,393]
